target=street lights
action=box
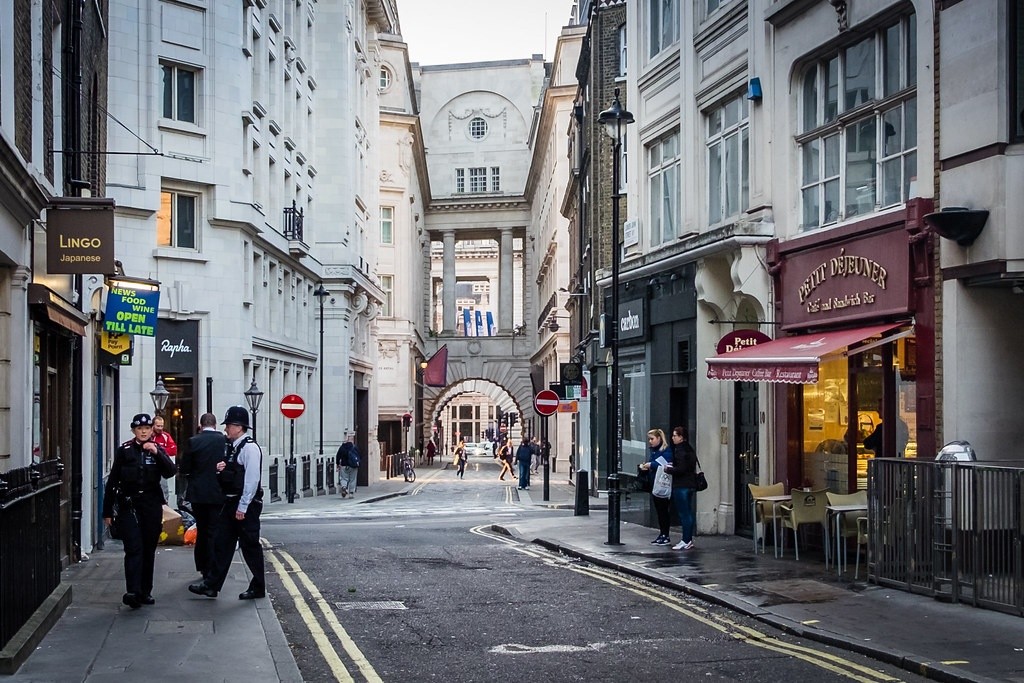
[596,87,636,546]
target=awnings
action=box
[704,320,915,385]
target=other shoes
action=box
[518,486,522,490]
[349,494,354,499]
[500,477,505,481]
[525,487,529,490]
[342,487,346,498]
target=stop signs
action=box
[280,394,305,419]
[534,390,560,416]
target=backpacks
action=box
[518,445,531,462]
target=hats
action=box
[131,413,152,428]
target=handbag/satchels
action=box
[347,444,360,468]
[110,502,123,540]
[695,471,707,491]
[633,464,651,492]
[652,464,674,500]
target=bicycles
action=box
[401,458,416,482]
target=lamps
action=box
[548,314,571,332]
[415,355,427,368]
[559,287,570,292]
[254,201,263,211]
[104,276,162,292]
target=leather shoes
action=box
[189,581,218,597]
[239,587,265,599]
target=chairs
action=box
[853,497,903,580]
[748,482,790,553]
[780,487,832,563]
[825,490,868,572]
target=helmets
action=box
[221,405,254,429]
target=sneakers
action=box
[123,593,141,610]
[672,539,693,550]
[650,534,670,546]
[143,595,156,605]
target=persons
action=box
[516,437,551,490]
[179,413,226,580]
[664,426,697,550]
[863,398,909,506]
[639,429,673,546]
[148,416,178,509]
[102,414,177,608]
[427,440,435,465]
[453,440,467,479]
[499,441,516,480]
[188,406,265,600]
[336,438,361,499]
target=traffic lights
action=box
[491,428,495,439]
[485,428,490,438]
[481,431,484,439]
[510,413,518,427]
[501,412,508,427]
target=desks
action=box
[825,505,868,575]
[751,495,792,560]
